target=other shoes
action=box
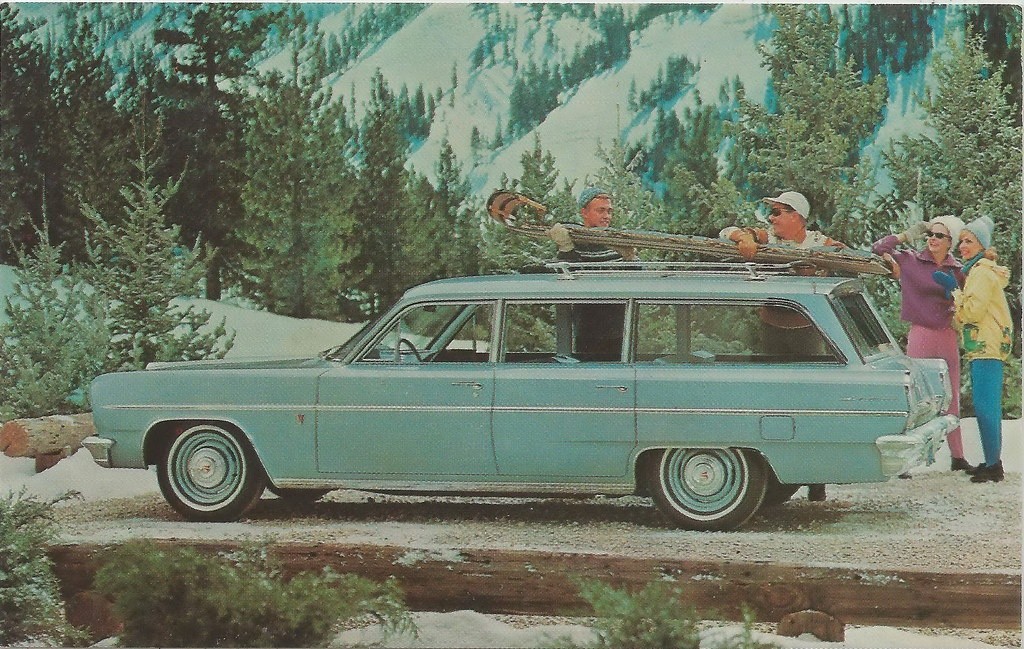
[808,484,826,502]
[898,472,912,480]
[951,457,974,471]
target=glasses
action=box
[926,230,951,239]
[769,208,793,217]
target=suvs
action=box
[82,261,961,532]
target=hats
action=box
[930,215,966,253]
[962,215,994,249]
[762,191,810,218]
[579,187,607,209]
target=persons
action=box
[551,187,643,501]
[870,215,975,481]
[934,213,1013,484]
[718,191,901,500]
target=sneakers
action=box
[970,463,1004,482]
[966,460,1003,474]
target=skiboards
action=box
[487,185,888,276]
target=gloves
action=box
[550,222,575,253]
[735,232,757,260]
[904,221,931,245]
[933,269,957,299]
[613,246,639,261]
[882,252,900,280]
